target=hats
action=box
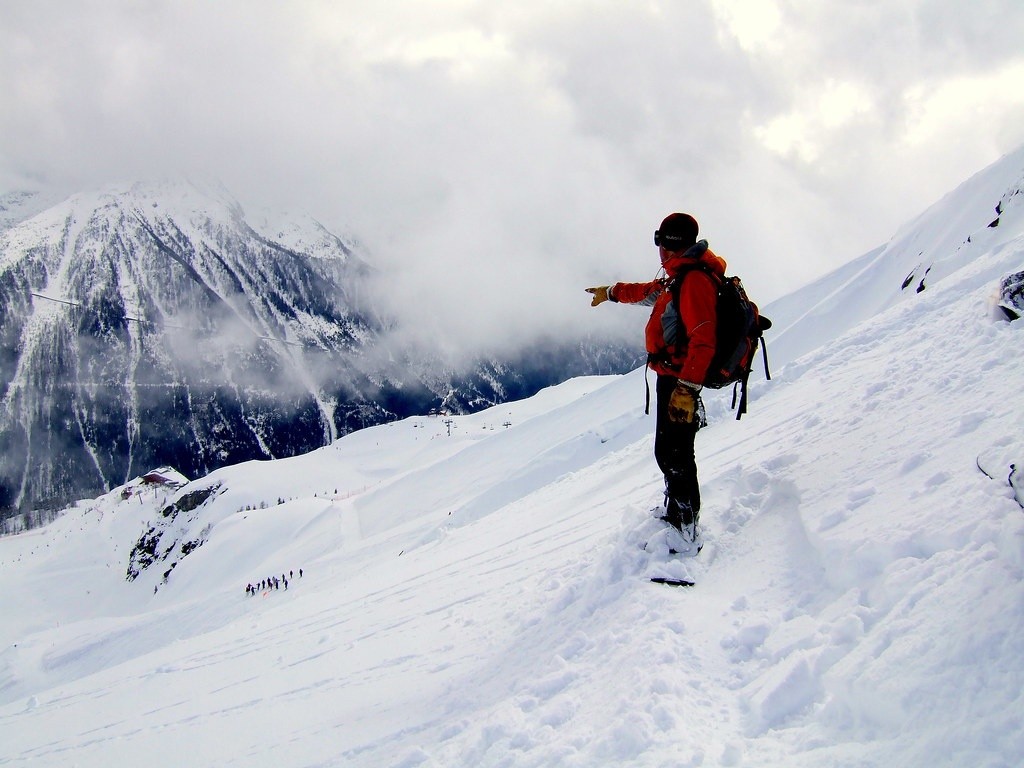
[658,212,699,250]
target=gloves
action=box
[585,285,618,306]
[668,382,699,424]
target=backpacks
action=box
[670,261,772,389]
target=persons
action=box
[585,213,727,561]
